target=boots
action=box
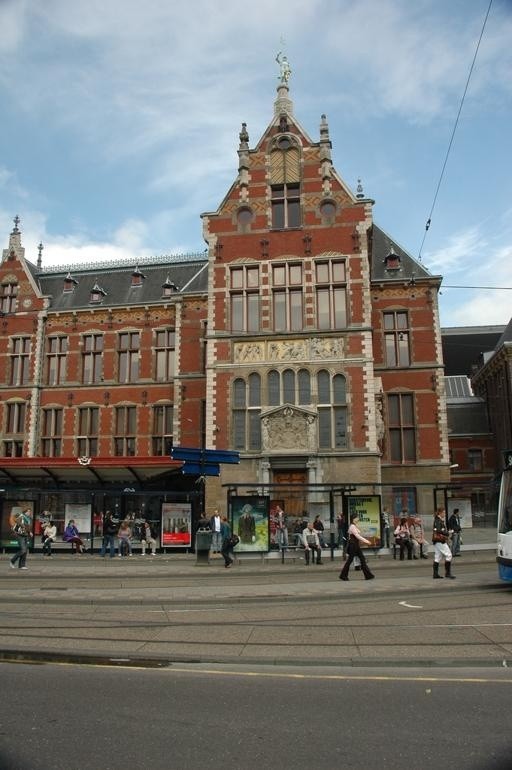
[444,560,457,579]
[432,561,444,579]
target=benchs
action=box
[278,544,316,565]
[118,538,151,554]
[46,540,76,555]
[390,541,422,561]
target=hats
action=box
[400,517,408,526]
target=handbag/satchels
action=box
[12,514,30,537]
[353,555,362,571]
[432,530,449,543]
[227,536,240,548]
[393,526,401,537]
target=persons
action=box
[220,516,233,568]
[63,520,89,556]
[381,506,429,561]
[10,508,34,569]
[238,504,256,545]
[273,504,328,565]
[336,512,343,548]
[196,511,212,530]
[340,513,375,580]
[448,509,462,556]
[40,521,57,556]
[92,509,158,558]
[40,508,52,542]
[211,508,221,554]
[432,508,456,578]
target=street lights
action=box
[473,487,483,514]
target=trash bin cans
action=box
[195,527,213,565]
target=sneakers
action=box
[18,566,30,570]
[9,560,17,570]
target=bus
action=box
[494,448,512,584]
